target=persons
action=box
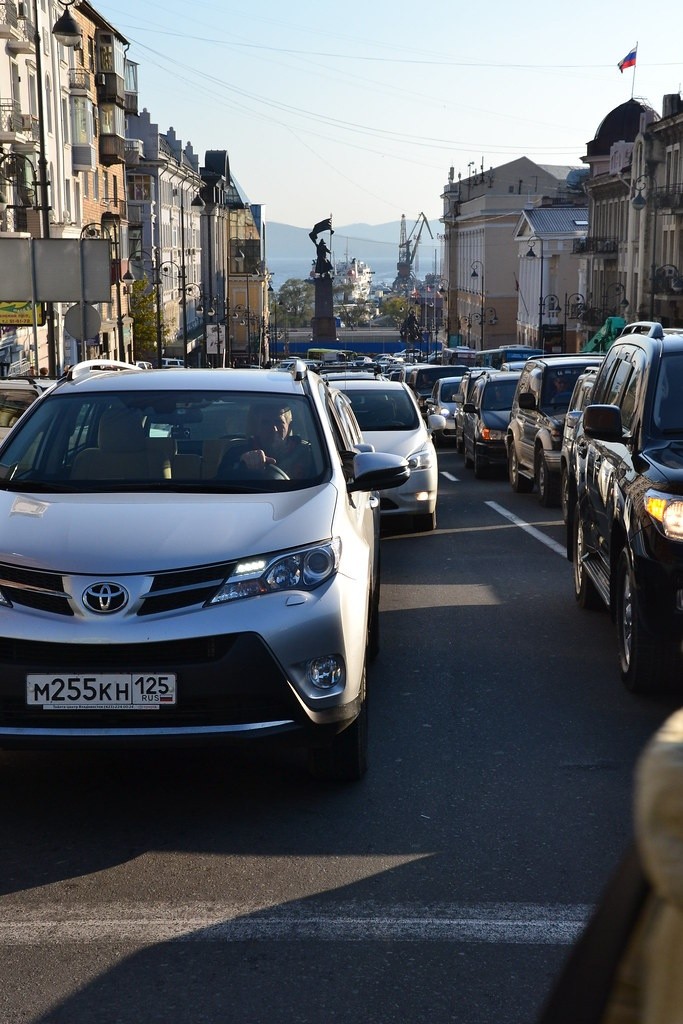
[311,239,332,277]
[217,404,315,480]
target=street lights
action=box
[439,279,450,347]
[601,282,630,325]
[564,292,588,352]
[457,312,484,347]
[470,261,499,351]
[525,236,560,349]
[80,176,285,369]
[0,0,83,381]
[631,174,683,322]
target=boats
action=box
[303,237,373,305]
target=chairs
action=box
[76,404,172,482]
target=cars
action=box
[161,358,190,369]
[426,376,464,448]
[136,360,153,370]
[324,381,447,533]
[1,375,89,476]
[276,349,527,429]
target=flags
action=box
[617,47,636,72]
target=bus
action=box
[475,344,545,370]
[441,346,477,367]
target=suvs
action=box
[505,353,608,509]
[463,370,524,480]
[0,359,412,797]
[560,366,600,525]
[567,321,683,694]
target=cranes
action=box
[392,212,434,291]
